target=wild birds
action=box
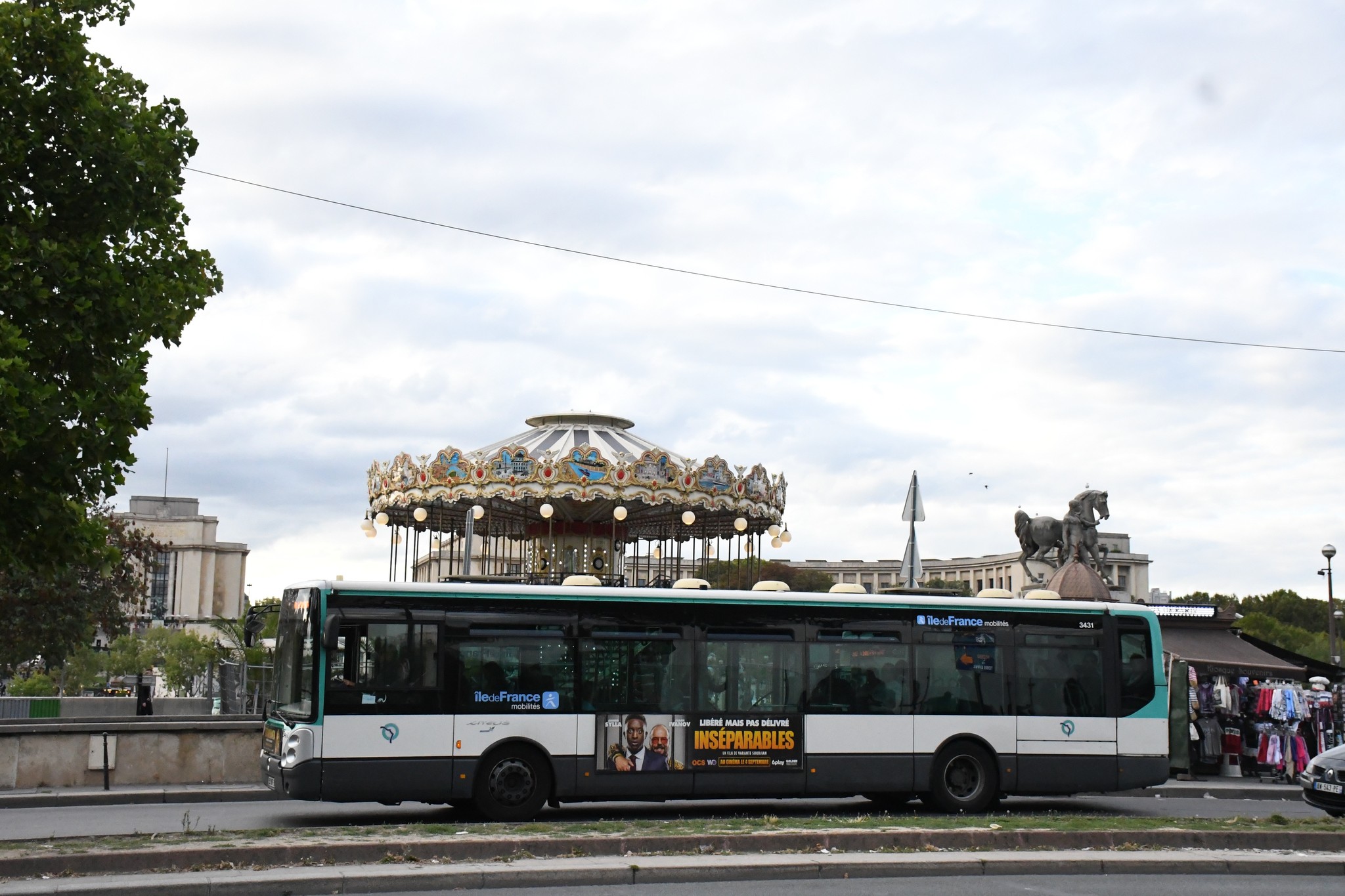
[969,473,973,475]
[984,485,989,489]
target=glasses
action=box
[651,737,668,744]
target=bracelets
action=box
[613,753,623,764]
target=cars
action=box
[1298,742,1345,819]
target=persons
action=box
[1062,500,1100,569]
[604,714,685,771]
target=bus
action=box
[245,579,1172,826]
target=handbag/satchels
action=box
[1189,723,1200,741]
[1212,675,1231,711]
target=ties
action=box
[630,755,638,771]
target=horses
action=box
[1014,490,1114,585]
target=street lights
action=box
[1319,543,1344,666]
